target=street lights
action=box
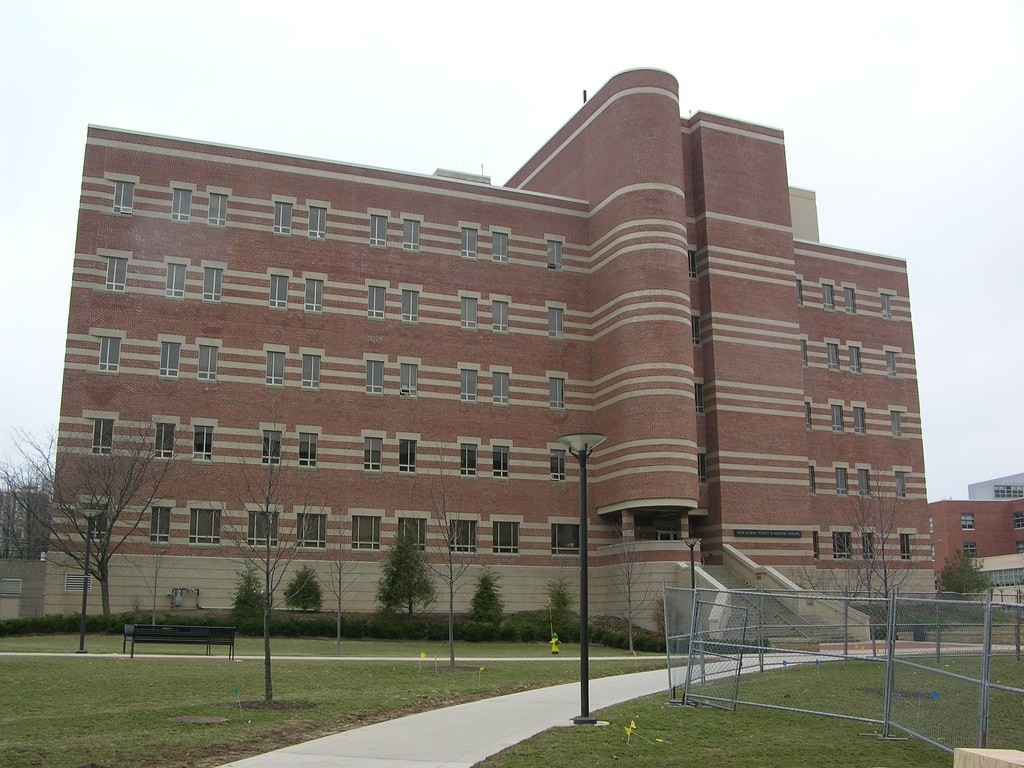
[683,537,702,610]
[556,432,608,725]
[75,508,104,653]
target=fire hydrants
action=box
[549,631,562,654]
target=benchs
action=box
[123,623,236,661]
